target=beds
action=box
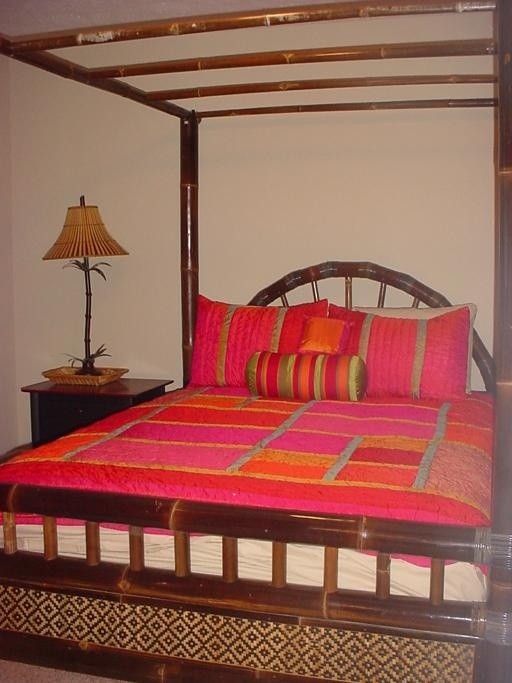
[2,12,511,683]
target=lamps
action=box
[43,195,130,383]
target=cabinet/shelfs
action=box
[19,379,175,447]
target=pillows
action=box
[180,295,474,401]
